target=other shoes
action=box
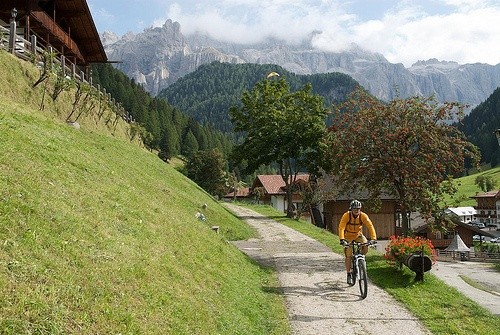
[346,273,352,284]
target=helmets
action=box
[350,200,362,209]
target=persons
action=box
[339,200,377,284]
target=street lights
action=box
[8,8,18,54]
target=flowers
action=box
[383,234,437,265]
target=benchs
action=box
[211,226,219,232]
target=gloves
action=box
[340,240,347,247]
[369,240,376,248]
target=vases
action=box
[404,253,432,273]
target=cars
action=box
[468,221,500,243]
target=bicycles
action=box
[340,241,377,299]
